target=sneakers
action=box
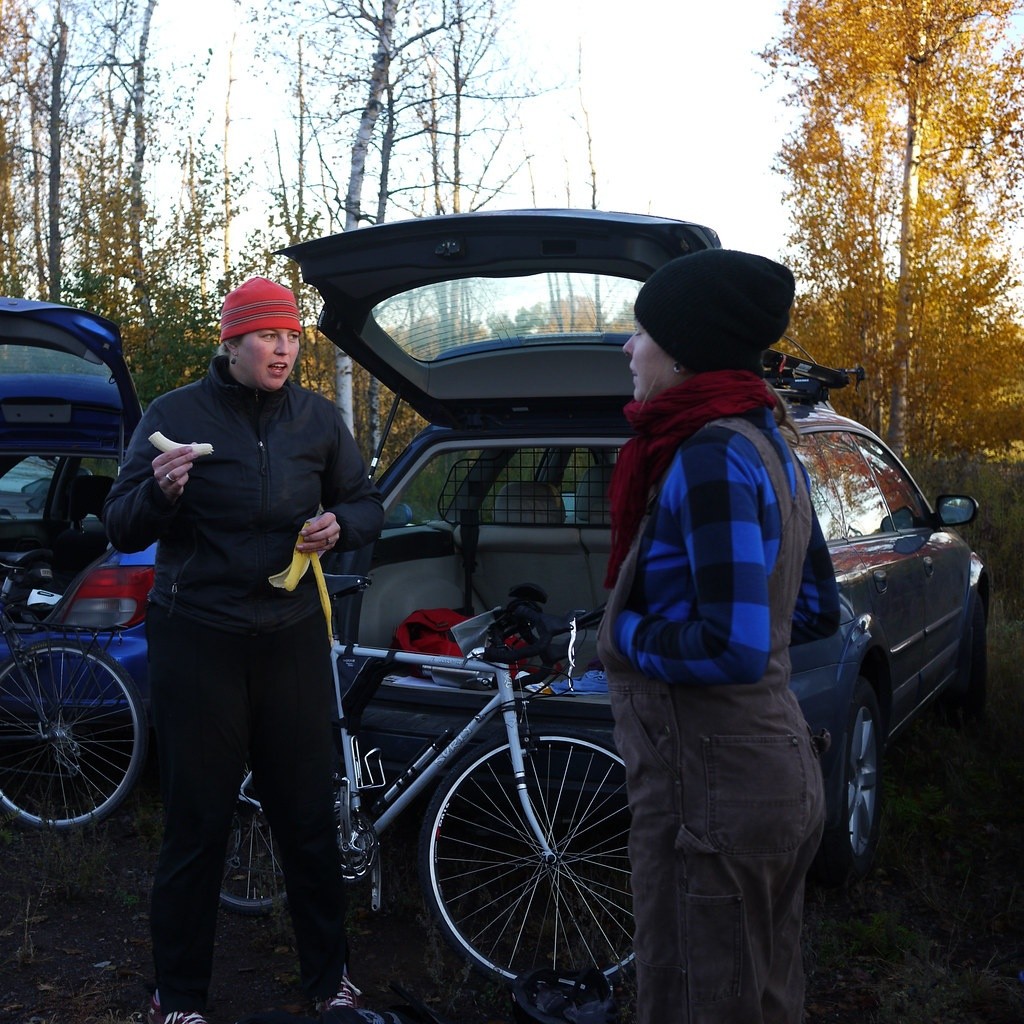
[310,1001,401,1024]
[147,990,208,1024]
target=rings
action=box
[166,474,175,481]
[325,537,330,546]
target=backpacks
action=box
[391,607,478,678]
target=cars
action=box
[0,297,160,724]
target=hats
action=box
[220,277,302,342]
[633,247,796,371]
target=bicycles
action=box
[217,572,637,995]
[1,547,147,835]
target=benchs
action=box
[454,463,619,625]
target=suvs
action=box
[271,207,989,894]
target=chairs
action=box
[59,474,113,561]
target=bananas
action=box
[147,431,214,455]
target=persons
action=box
[106,277,385,1024]
[597,249,837,1024]
